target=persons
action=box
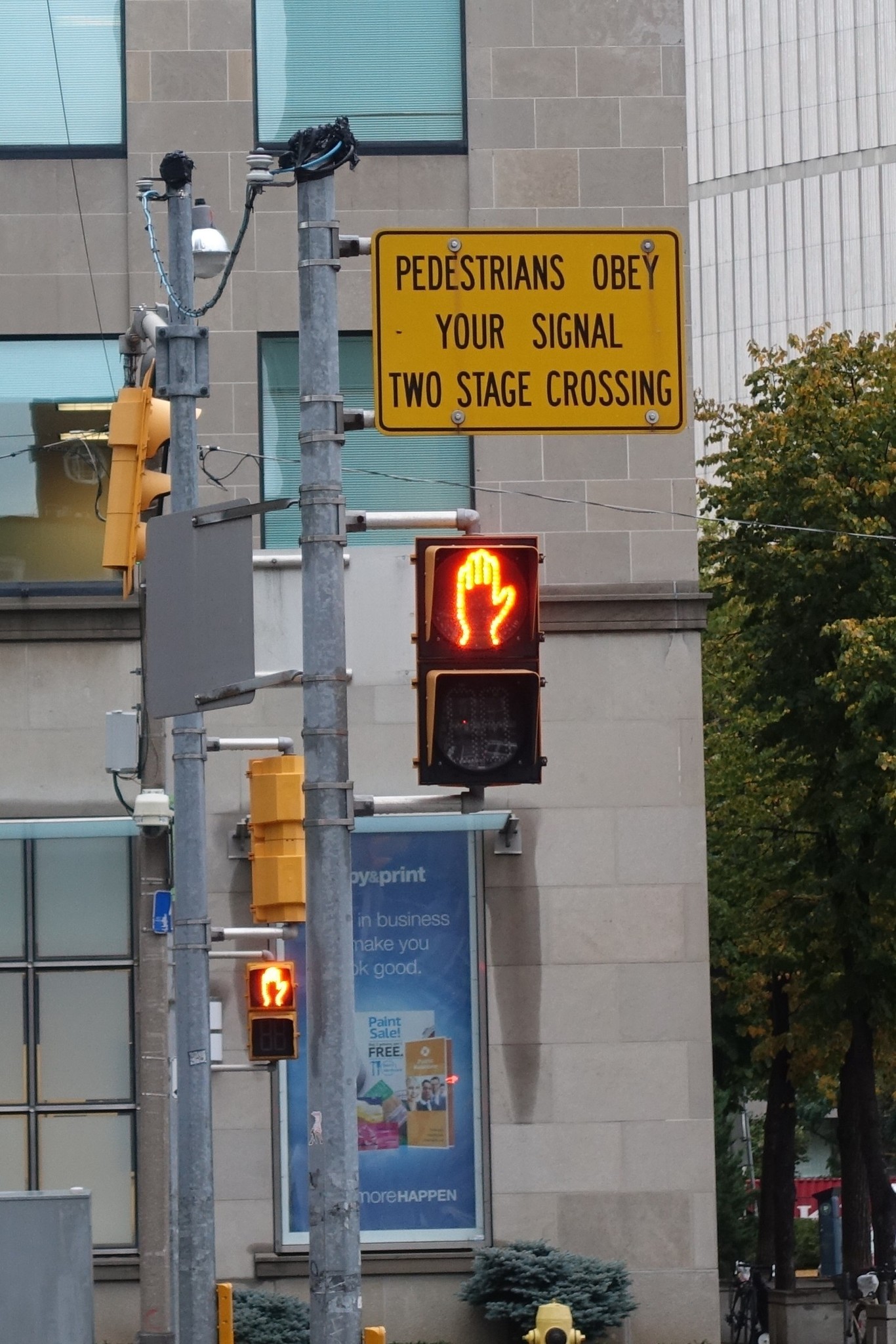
[405,1073,447,1112]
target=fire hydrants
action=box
[522,1297,585,1343]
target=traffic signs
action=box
[56,381,202,603]
[407,519,553,791]
[371,223,688,435]
[241,961,298,1066]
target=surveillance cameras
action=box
[132,788,171,838]
